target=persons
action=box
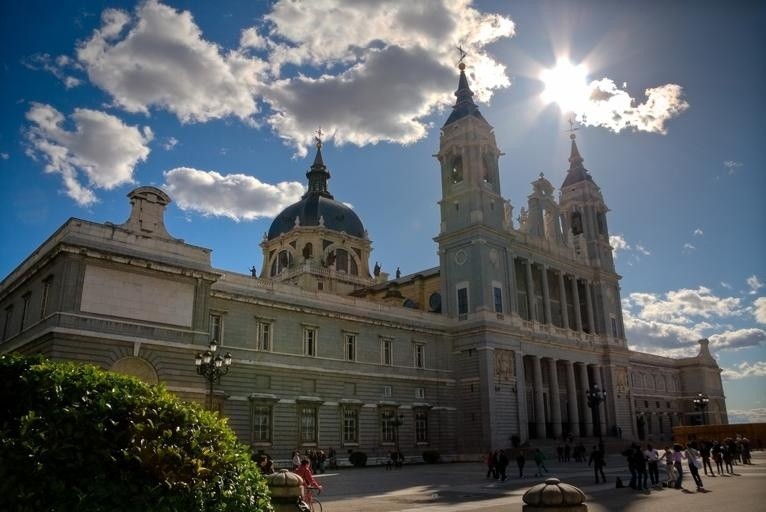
[385,448,392,470]
[250,445,335,503]
[390,452,404,469]
[486,433,752,491]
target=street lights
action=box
[391,413,405,462]
[692,392,710,424]
[587,382,607,430]
[196,338,232,414]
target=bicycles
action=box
[302,485,322,512]
